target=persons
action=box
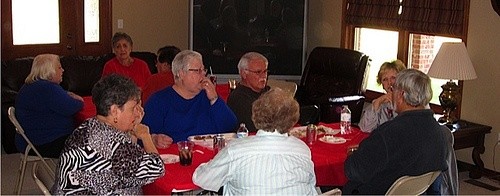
[137,50,239,150]
[192,86,318,196]
[359,59,430,133]
[340,68,453,196]
[227,52,271,133]
[101,32,152,92]
[14,54,84,159]
[141,47,181,109]
[55,73,165,195]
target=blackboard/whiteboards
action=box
[189,0,309,80]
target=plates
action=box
[288,126,307,138]
[190,133,216,142]
[319,136,346,144]
[160,154,179,164]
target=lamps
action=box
[427,43,477,124]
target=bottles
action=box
[340,105,352,135]
[237,124,248,138]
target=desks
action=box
[143,120,371,196]
[75,85,229,124]
[433,115,492,179]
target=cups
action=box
[177,141,195,165]
[228,79,239,92]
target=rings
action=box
[163,146,165,149]
[205,83,208,86]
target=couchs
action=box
[1,52,158,154]
[294,47,368,126]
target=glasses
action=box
[188,68,208,75]
[245,69,270,76]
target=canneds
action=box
[306,124,317,143]
[214,134,226,154]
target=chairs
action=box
[267,79,298,97]
[385,171,441,196]
[32,161,55,196]
[8,106,56,196]
